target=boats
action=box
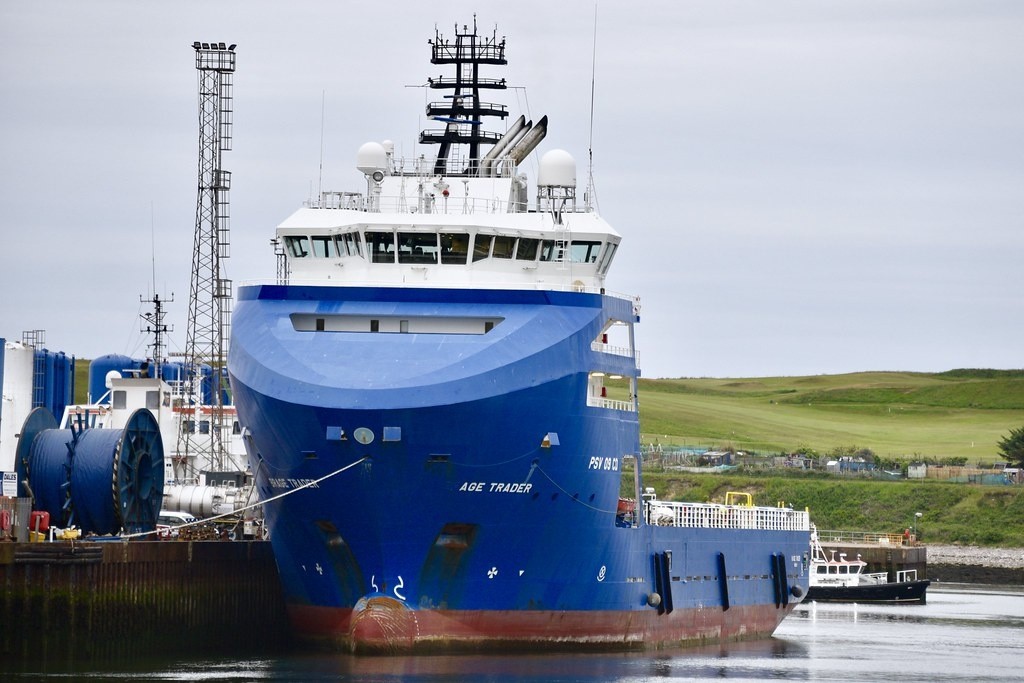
[801,521,931,604]
[228,10,822,655]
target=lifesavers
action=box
[843,590,850,597]
[909,534,915,542]
[861,589,870,597]
[876,589,885,597]
[823,589,831,598]
[1,509,11,530]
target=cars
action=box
[158,512,219,535]
